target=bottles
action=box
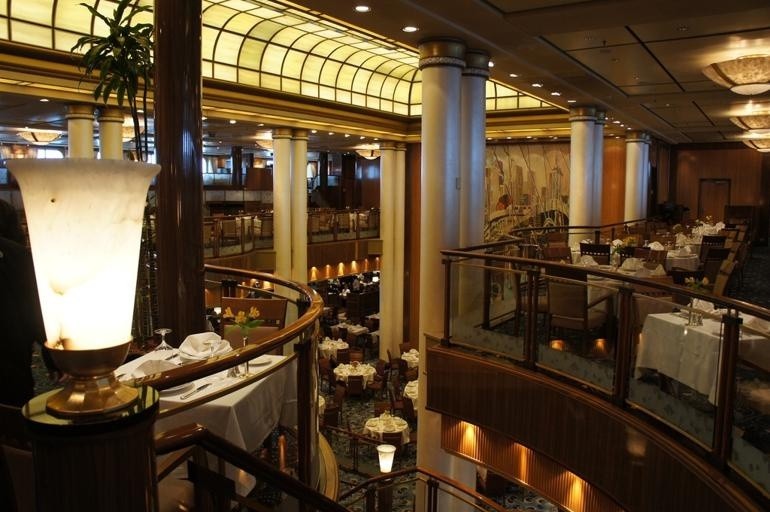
[690,312,703,326]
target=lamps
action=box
[17,128,61,147]
[1,158,162,420]
[355,148,380,161]
[377,443,396,486]
[699,56,770,153]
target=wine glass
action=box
[150,328,178,362]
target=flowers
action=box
[222,305,266,340]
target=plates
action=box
[368,417,404,432]
[587,276,605,280]
[246,354,271,366]
[407,383,416,387]
[678,255,691,258]
[158,381,193,394]
[624,268,638,272]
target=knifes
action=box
[179,383,209,400]
[116,374,126,378]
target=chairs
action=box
[204,203,380,246]
[317,271,421,460]
[219,296,290,355]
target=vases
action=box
[239,336,253,378]
